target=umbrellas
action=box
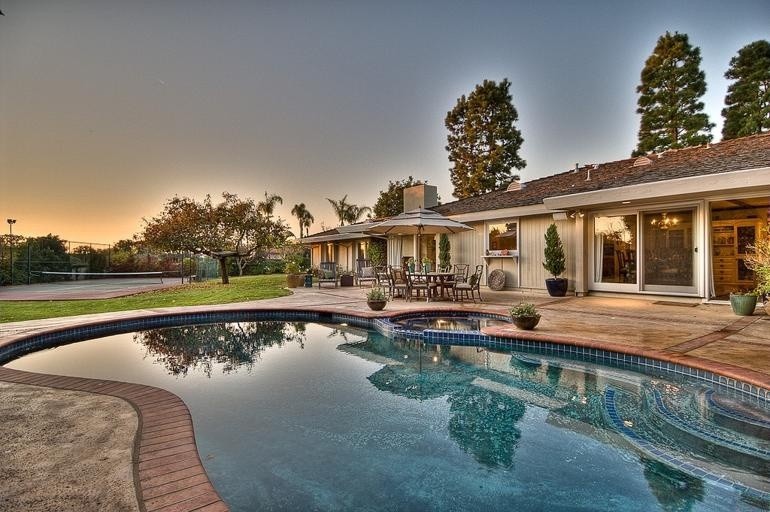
[367,204,475,273]
[365,340,478,403]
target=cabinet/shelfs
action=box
[712,217,763,291]
[666,221,692,248]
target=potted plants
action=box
[543,224,568,296]
[287,262,304,288]
[366,289,388,310]
[509,302,540,329]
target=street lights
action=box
[8,219,16,285]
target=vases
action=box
[730,291,757,316]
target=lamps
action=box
[578,209,584,218]
[650,213,679,230]
[570,209,581,218]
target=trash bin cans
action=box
[304,274,313,287]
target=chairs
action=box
[615,250,636,283]
[358,267,376,288]
[374,263,483,305]
[318,261,338,289]
